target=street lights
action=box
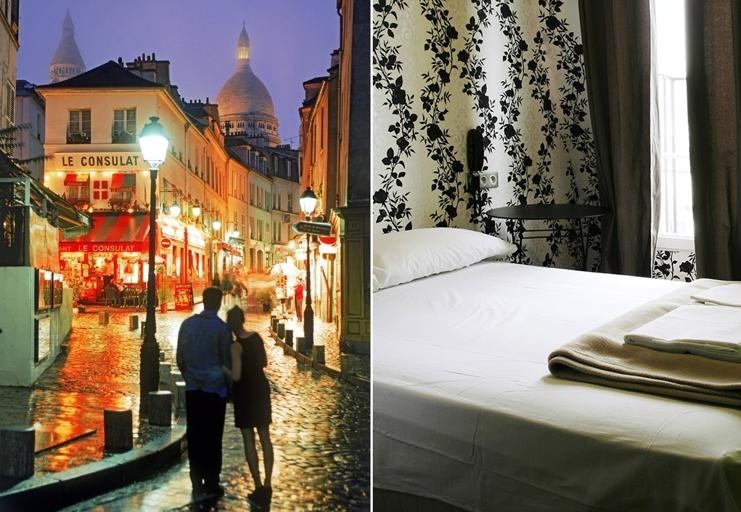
[138,114,170,420]
[298,186,317,349]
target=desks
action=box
[486,203,611,271]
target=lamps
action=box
[188,196,200,217]
[160,189,181,218]
[206,210,221,231]
[226,221,239,238]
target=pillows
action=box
[374,227,519,289]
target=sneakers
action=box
[190,480,225,503]
[247,486,272,502]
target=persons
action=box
[176,286,274,500]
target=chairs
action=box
[104,285,157,309]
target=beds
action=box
[371,258,741,512]
